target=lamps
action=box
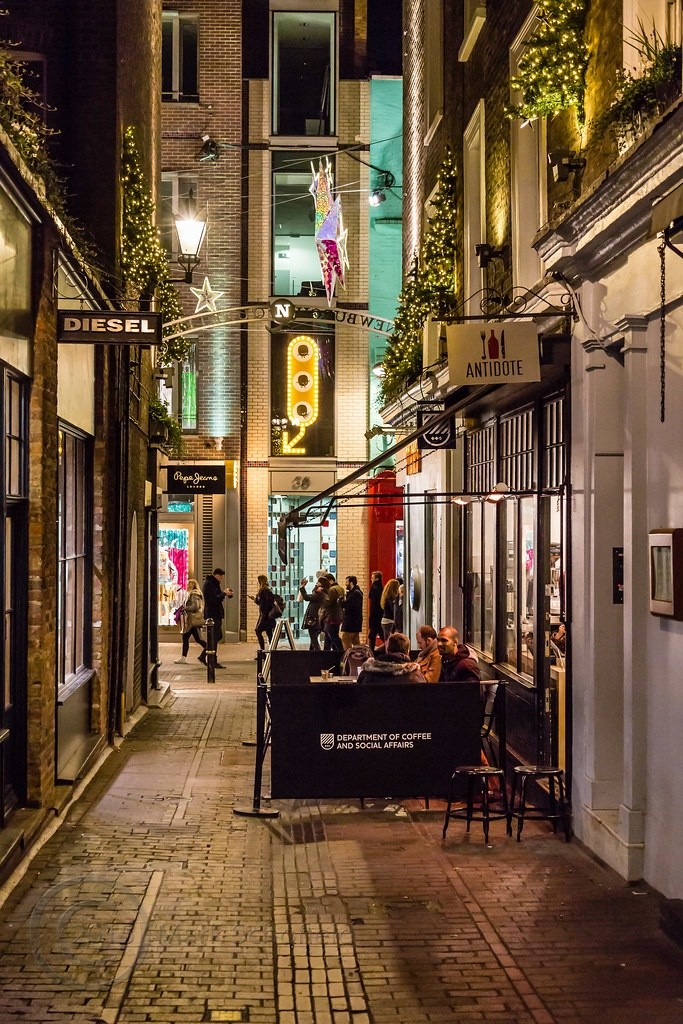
[369,188,386,207]
[163,188,207,284]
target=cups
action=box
[320,669,329,680]
[357,666,363,676]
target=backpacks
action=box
[333,587,346,622]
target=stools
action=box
[443,765,509,843]
[509,766,571,843]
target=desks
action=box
[310,676,357,686]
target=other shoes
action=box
[173,657,187,663]
[216,663,226,669]
[198,657,207,666]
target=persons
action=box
[173,579,206,664]
[414,625,442,683]
[523,624,567,662]
[299,567,405,651]
[249,575,276,660]
[197,567,233,669]
[356,633,427,684]
[436,626,485,727]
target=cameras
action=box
[227,589,234,599]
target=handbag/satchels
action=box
[340,647,373,676]
[268,594,285,619]
[191,612,204,626]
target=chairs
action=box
[340,646,373,676]
